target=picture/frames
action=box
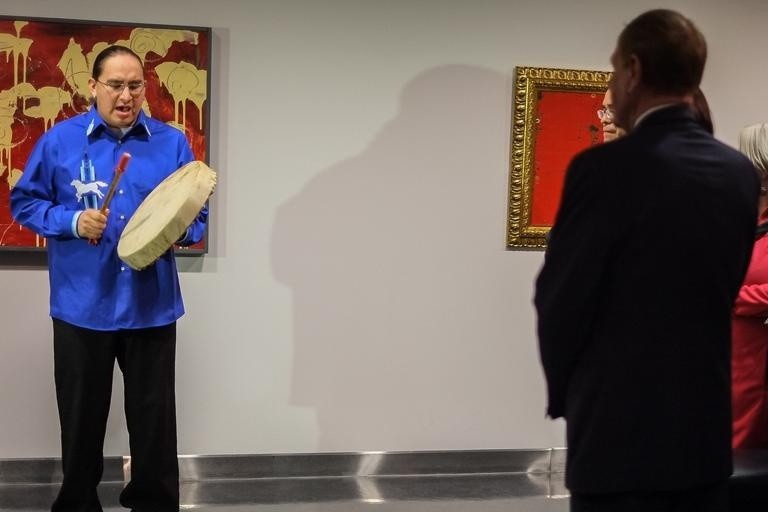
[0,16,216,260]
[504,62,622,253]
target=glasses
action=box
[93,79,145,92]
[596,109,618,121]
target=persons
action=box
[688,84,714,141]
[598,83,629,144]
[729,122,768,450]
[9,46,208,510]
[529,6,764,510]
